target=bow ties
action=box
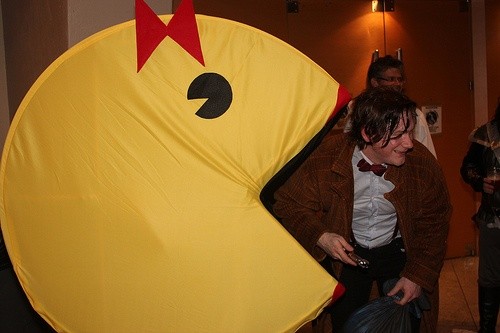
[357,157,388,177]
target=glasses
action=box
[376,76,402,82]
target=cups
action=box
[487,166,500,187]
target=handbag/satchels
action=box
[344,294,420,333]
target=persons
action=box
[343,56,438,159]
[272,86,453,333]
[460,98,500,333]
